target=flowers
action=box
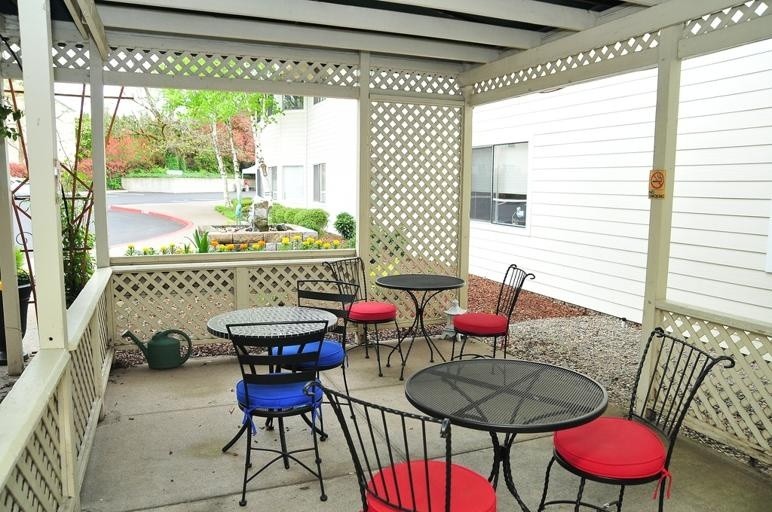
[125,235,339,255]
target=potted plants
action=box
[0,245,32,366]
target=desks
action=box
[206,306,338,470]
